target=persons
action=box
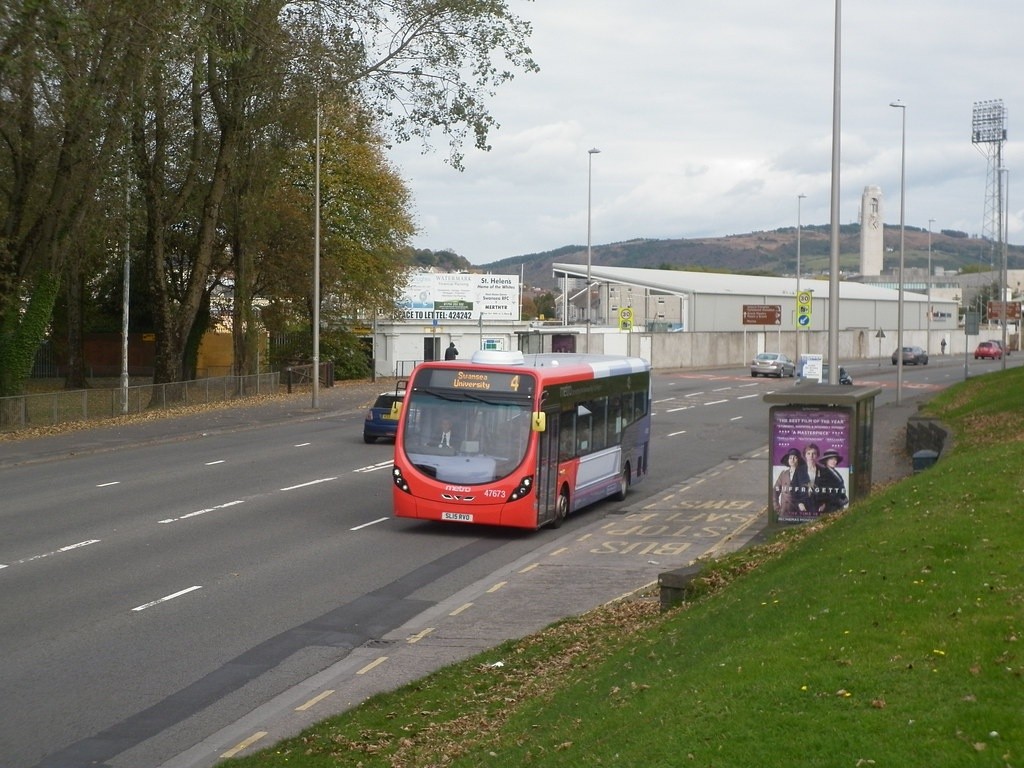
[445,343,458,360]
[424,416,461,449]
[791,444,829,512]
[818,449,849,513]
[559,429,572,460]
[772,448,805,516]
[941,338,946,354]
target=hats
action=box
[818,450,843,464]
[781,448,805,467]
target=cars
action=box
[363,390,421,445]
[988,340,1012,356]
[974,341,1003,360]
[751,352,796,379]
[892,345,928,366]
[794,363,853,388]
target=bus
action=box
[389,349,654,530]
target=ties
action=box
[442,433,447,449]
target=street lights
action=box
[586,147,602,354]
[890,98,906,406]
[927,218,937,358]
[795,195,806,363]
[996,165,1011,371]
